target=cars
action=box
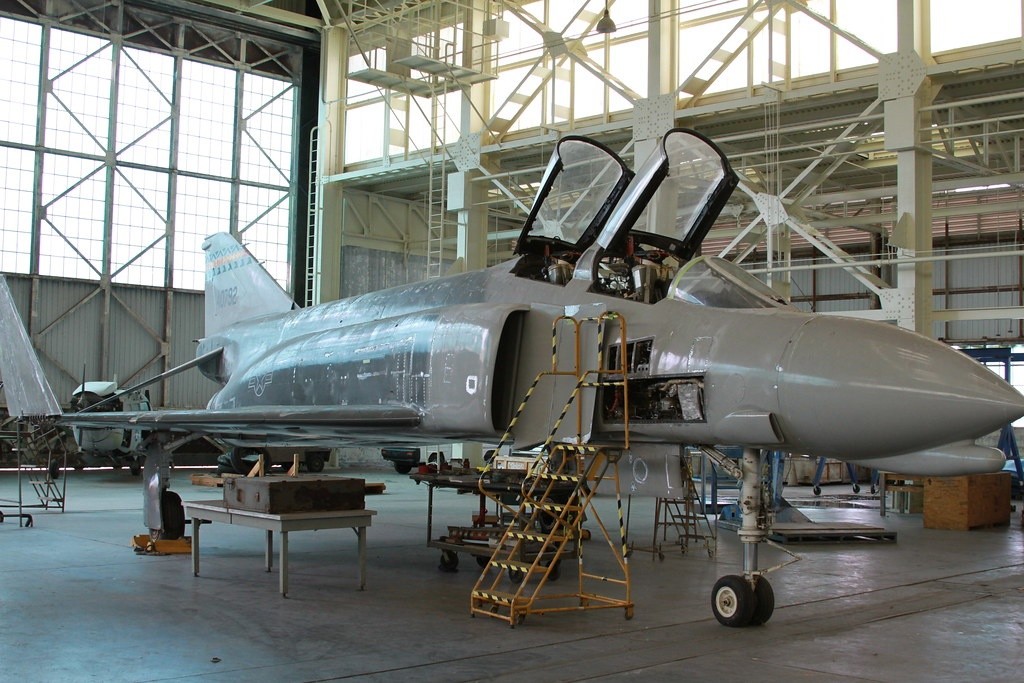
[1002,459,1024,497]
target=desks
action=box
[181,499,379,601]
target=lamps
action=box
[595,0,616,33]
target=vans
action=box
[381,443,499,473]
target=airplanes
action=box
[4,362,192,481]
[0,131,1024,624]
